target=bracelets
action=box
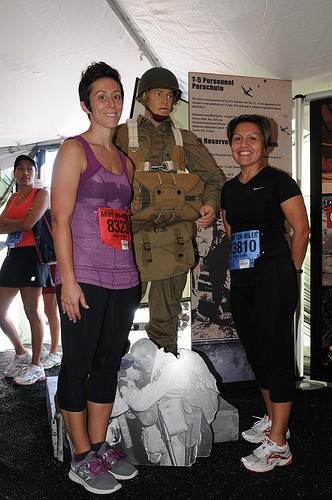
[296,268,304,273]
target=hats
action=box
[14,154,36,172]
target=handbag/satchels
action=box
[31,209,58,265]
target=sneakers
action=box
[2,349,33,377]
[240,435,292,472]
[40,351,62,369]
[242,415,289,443]
[13,363,47,385]
[97,441,138,480]
[28,345,48,364]
[69,451,122,494]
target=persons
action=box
[50,62,141,494]
[221,116,312,472]
[0,151,48,386]
[36,200,65,371]
[113,66,227,354]
